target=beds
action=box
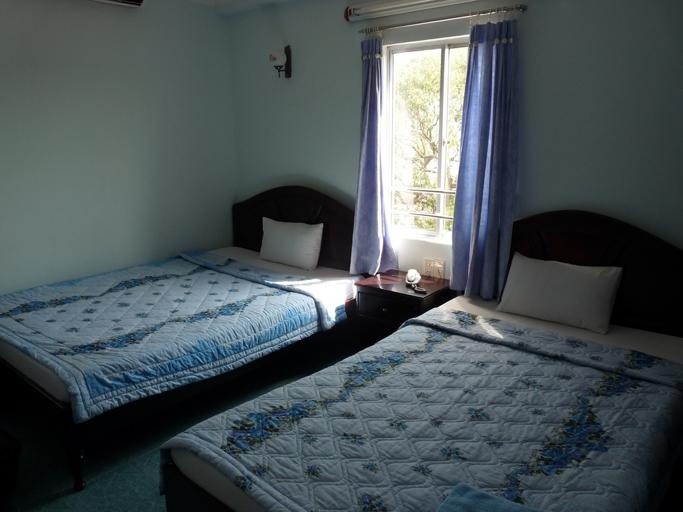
[159,210,683,508]
[2,183,360,488]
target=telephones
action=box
[405,269,422,287]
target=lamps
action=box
[265,33,293,82]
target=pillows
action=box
[496,250,621,335]
[258,216,324,272]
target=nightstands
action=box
[353,269,455,338]
